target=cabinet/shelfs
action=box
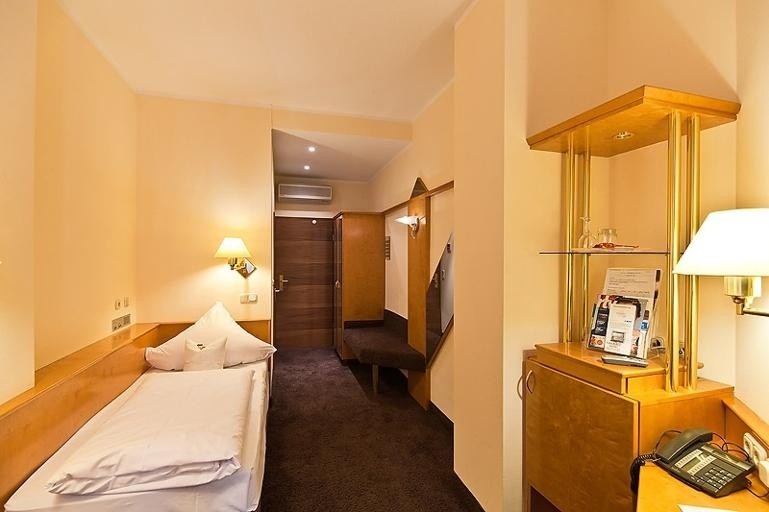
[332,212,386,364]
[519,84,741,511]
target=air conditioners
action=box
[277,182,333,204]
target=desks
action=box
[637,395,768,512]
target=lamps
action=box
[213,237,256,279]
[673,207,769,315]
[395,216,417,240]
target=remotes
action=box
[600,355,649,368]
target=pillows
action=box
[182,337,227,371]
[145,301,277,370]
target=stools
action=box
[344,324,427,394]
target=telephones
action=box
[653,427,755,497]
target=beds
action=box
[3,301,277,511]
